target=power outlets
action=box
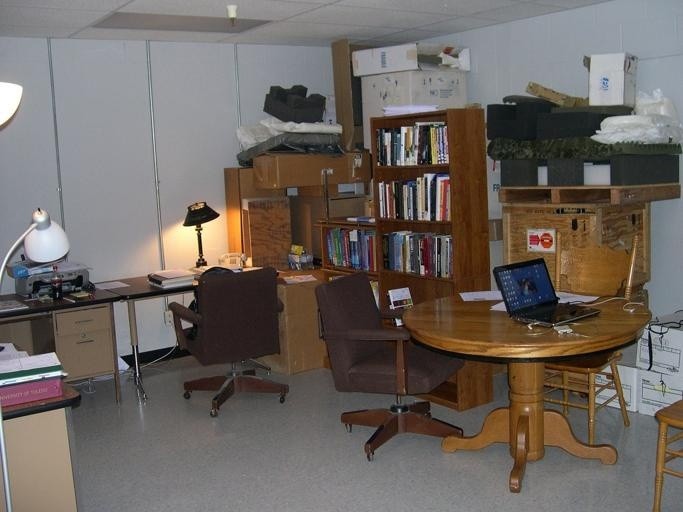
[165,309,175,327]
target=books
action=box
[326,122,451,278]
[146,279,192,289]
[0,376,64,408]
[0,351,63,387]
[147,270,192,285]
[386,287,413,326]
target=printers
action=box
[15,266,89,299]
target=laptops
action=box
[493,256,601,326]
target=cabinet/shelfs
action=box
[224,108,495,412]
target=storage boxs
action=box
[361,72,468,150]
[500,204,650,296]
[583,52,637,109]
[591,311,682,416]
[351,39,471,77]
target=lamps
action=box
[1,81,23,127]
[0,209,70,285]
[184,202,219,267]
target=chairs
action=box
[314,272,463,461]
[545,231,640,448]
[652,399,683,512]
[169,266,290,417]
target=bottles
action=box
[51,265,64,303]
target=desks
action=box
[1,261,254,405]
[0,341,82,512]
[400,287,651,494]
